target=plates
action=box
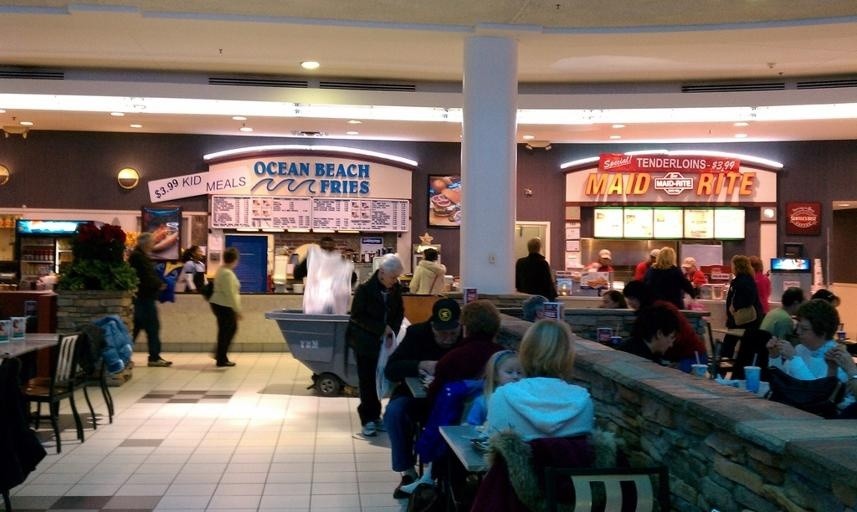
[471,436,491,453]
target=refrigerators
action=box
[15,218,94,291]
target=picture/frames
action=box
[141,204,182,261]
[428,173,462,230]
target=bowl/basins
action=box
[292,283,304,293]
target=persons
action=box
[581,248,857,419]
[181,246,205,294]
[208,247,243,366]
[515,238,558,302]
[127,233,172,367]
[346,253,404,437]
[384,298,595,499]
[408,248,446,294]
[294,237,357,283]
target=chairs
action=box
[0,354,46,512]
[29,317,119,430]
[695,320,731,375]
[438,380,485,426]
[25,334,84,453]
[481,431,588,511]
[553,464,669,511]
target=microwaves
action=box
[360,237,384,250]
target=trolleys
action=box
[264,309,360,397]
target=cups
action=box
[12,317,26,339]
[692,364,706,374]
[1,319,12,343]
[722,290,727,300]
[837,331,846,339]
[714,286,721,297]
[611,336,624,342]
[745,365,761,393]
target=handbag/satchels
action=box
[200,281,213,302]
[732,303,757,327]
[407,482,454,512]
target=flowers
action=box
[59,221,138,291]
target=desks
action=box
[439,424,496,474]
[406,375,428,401]
[1,334,64,392]
[713,326,749,344]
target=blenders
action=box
[272,256,287,293]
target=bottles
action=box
[342,247,394,262]
[24,263,53,276]
[22,248,54,261]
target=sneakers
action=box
[148,358,173,368]
[216,359,236,367]
[393,473,417,500]
[362,421,379,437]
[375,416,388,433]
[400,476,434,495]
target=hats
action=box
[649,249,660,258]
[596,249,613,260]
[680,258,696,268]
[432,299,462,330]
